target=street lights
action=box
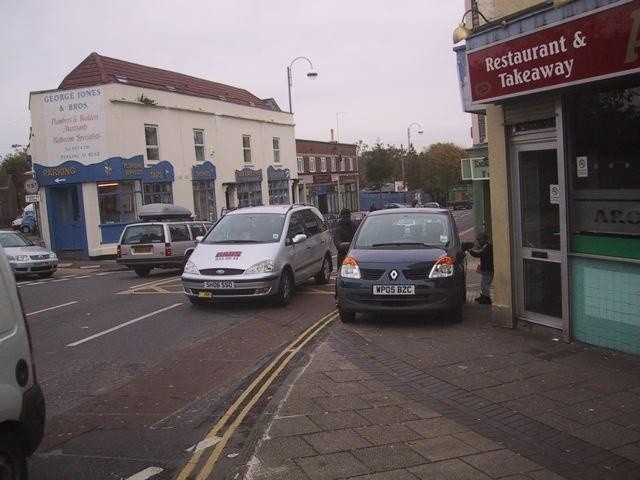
[401,154,408,180]
[407,123,423,153]
[286,56,317,112]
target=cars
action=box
[19,211,36,233]
[335,199,475,323]
[0,230,58,279]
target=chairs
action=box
[392,223,443,244]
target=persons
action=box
[334,208,358,309]
[412,198,418,207]
[369,204,377,212]
[467,233,494,304]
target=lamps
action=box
[452,9,507,44]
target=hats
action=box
[341,209,352,217]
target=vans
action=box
[0,244,46,479]
[11,203,36,229]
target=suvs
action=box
[183,204,334,306]
[116,220,213,277]
